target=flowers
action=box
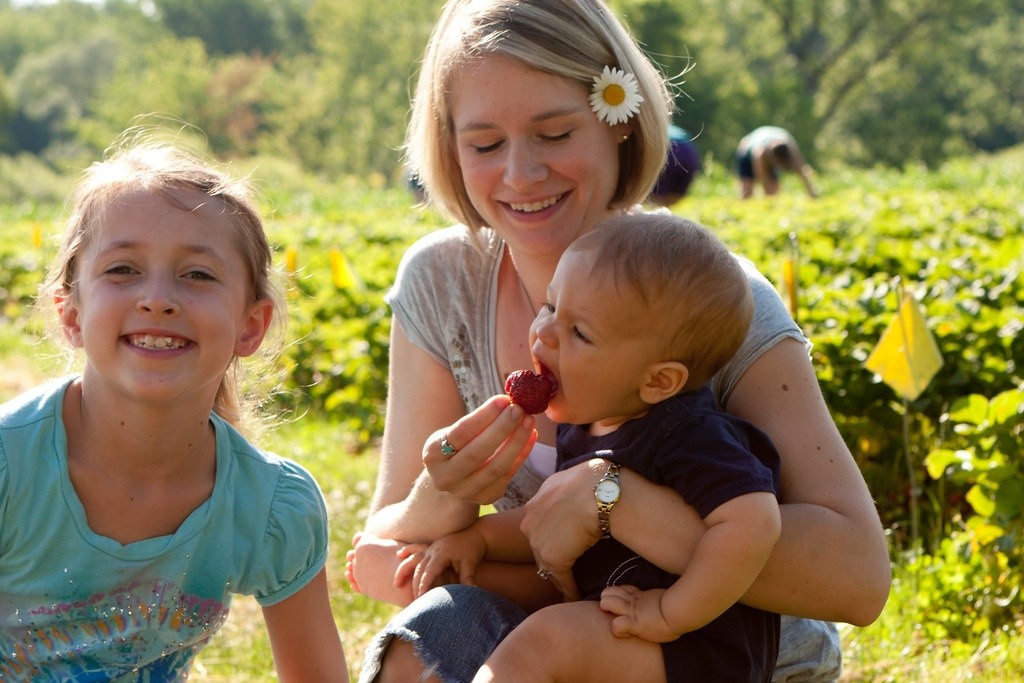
[587,64,646,127]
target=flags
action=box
[864,292,946,402]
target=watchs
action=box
[594,460,625,539]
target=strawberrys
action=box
[505,370,554,413]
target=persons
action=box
[365,0,891,683]
[737,126,821,199]
[344,215,788,683]
[640,123,704,208]
[0,145,350,683]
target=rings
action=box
[537,569,553,584]
[441,427,456,457]
